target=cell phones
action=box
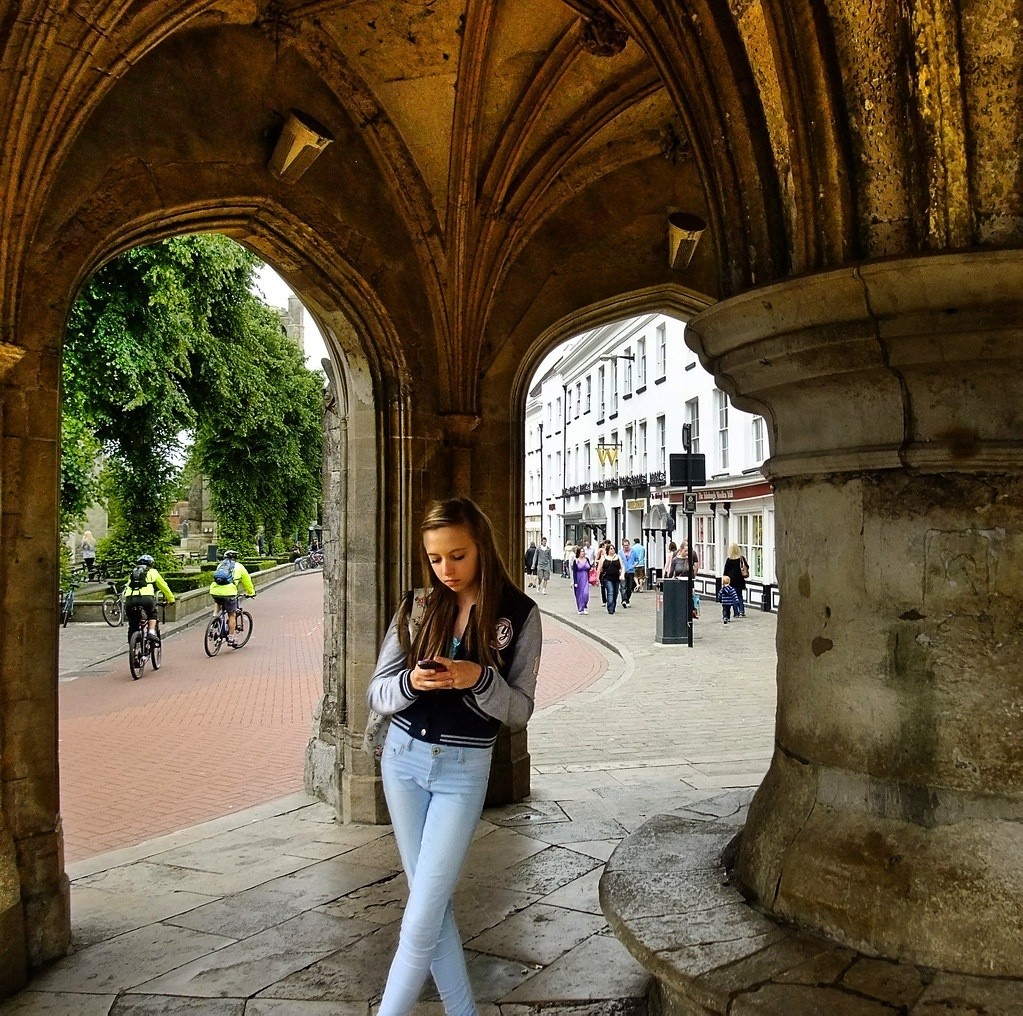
[418,660,447,674]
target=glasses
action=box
[610,548,615,550]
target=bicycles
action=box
[302,550,324,570]
[61,579,89,628]
[71,560,111,585]
[102,580,127,626]
[129,601,170,679]
[204,594,256,657]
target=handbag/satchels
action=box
[740,556,749,578]
[361,588,435,760]
[599,572,606,582]
[589,562,599,583]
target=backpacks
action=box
[213,558,236,585]
[129,565,154,588]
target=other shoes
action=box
[528,583,533,587]
[578,611,583,614]
[639,588,643,592]
[533,585,536,588]
[734,615,739,618]
[212,622,218,629]
[134,659,140,667]
[622,603,627,608]
[228,638,238,646]
[536,587,540,593]
[584,608,588,615]
[724,619,727,624]
[626,604,631,608]
[543,588,546,594]
[740,612,745,616]
[147,633,159,642]
[602,603,606,607]
[634,585,640,592]
[728,619,730,622]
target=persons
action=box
[526,542,538,588]
[366,495,542,1016]
[125,555,176,656]
[563,540,625,616]
[618,540,640,609]
[718,576,739,624]
[631,538,645,592]
[723,543,749,617]
[664,539,698,596]
[311,541,318,553]
[289,546,305,571]
[209,551,256,645]
[81,531,96,567]
[531,537,552,594]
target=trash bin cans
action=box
[207,544,218,562]
[654,578,689,644]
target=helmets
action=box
[223,550,239,559]
[137,555,154,566]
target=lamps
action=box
[268,108,333,186]
[668,210,705,271]
[601,352,635,363]
